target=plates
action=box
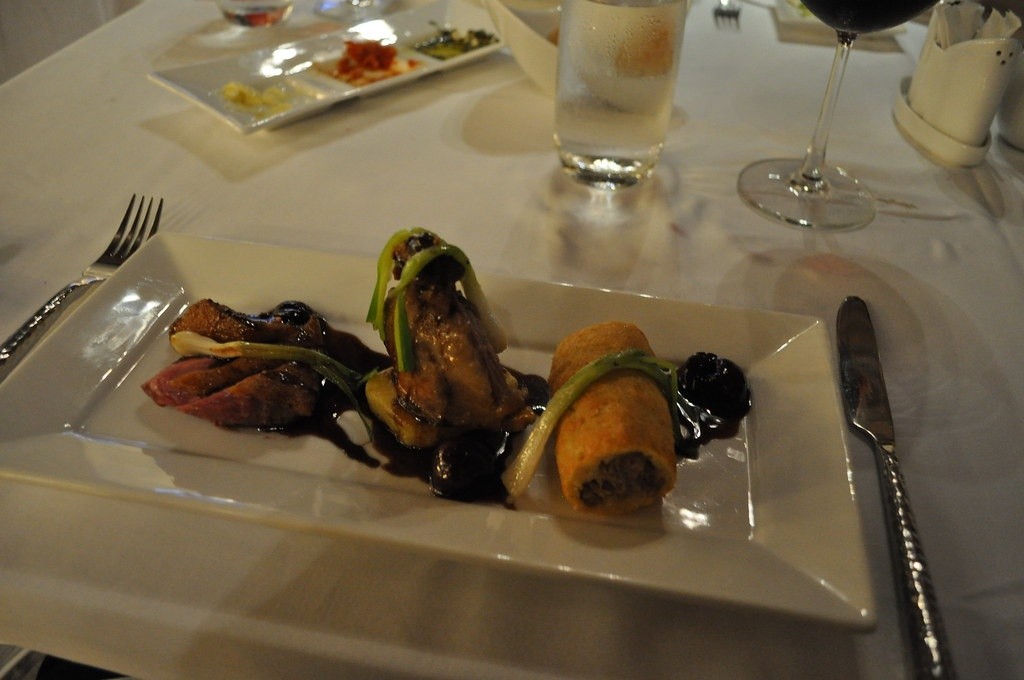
[146,0,506,134]
[0,230,876,627]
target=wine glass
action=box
[739,0,946,227]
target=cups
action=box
[214,0,294,28]
[551,0,695,192]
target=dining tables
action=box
[1,0,1024,680]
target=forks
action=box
[713,0,740,30]
[0,193,164,365]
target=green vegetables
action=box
[168,230,683,501]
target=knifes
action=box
[835,295,954,679]
